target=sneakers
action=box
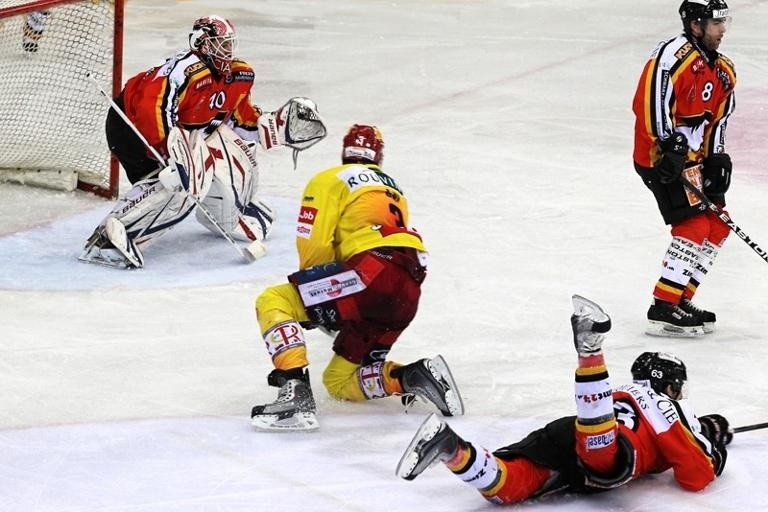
[84,225,124,261]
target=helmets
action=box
[679,0,728,36]
[342,125,383,167]
[188,14,237,75]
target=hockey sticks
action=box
[84,71,268,263]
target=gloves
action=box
[703,153,730,196]
[655,132,685,184]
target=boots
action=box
[405,422,462,480]
[389,357,450,417]
[250,371,317,417]
[570,313,610,355]
[646,296,717,325]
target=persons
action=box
[394,293,738,509]
[628,0,739,340]
[17,2,56,59]
[250,122,466,431]
[79,15,327,271]
